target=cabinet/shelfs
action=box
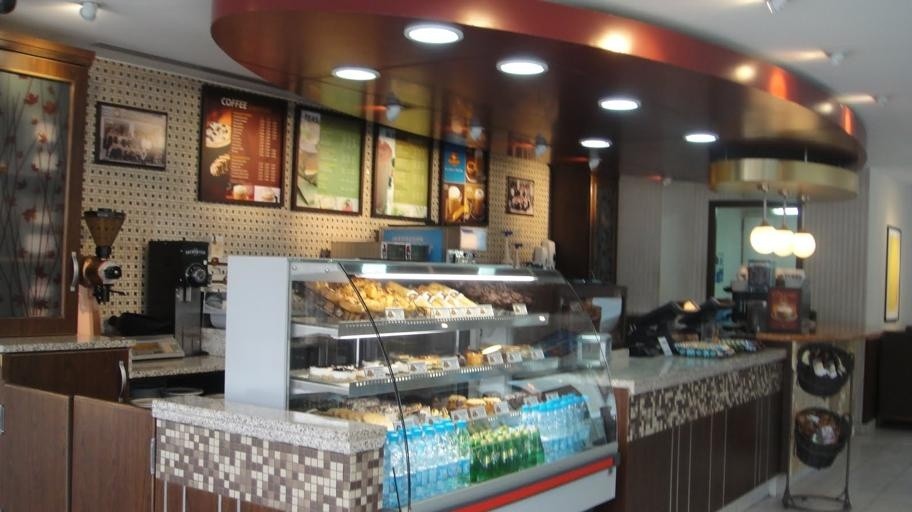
[0,348,129,403]
[0,29,96,338]
[224,255,620,512]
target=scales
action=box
[112,333,186,360]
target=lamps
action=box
[750,184,816,259]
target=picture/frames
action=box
[199,84,288,209]
[370,122,434,222]
[884,224,902,322]
[94,101,168,171]
[439,140,490,227]
[505,176,535,217]
[291,103,367,217]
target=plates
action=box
[130,385,205,409]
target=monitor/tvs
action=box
[748,261,772,291]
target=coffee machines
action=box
[77,207,127,338]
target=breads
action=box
[313,394,449,433]
[452,280,534,309]
[305,277,419,314]
[466,345,533,366]
[304,352,444,384]
[432,391,536,418]
[379,280,480,309]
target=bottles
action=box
[384,418,470,508]
[519,393,591,462]
[468,423,545,483]
